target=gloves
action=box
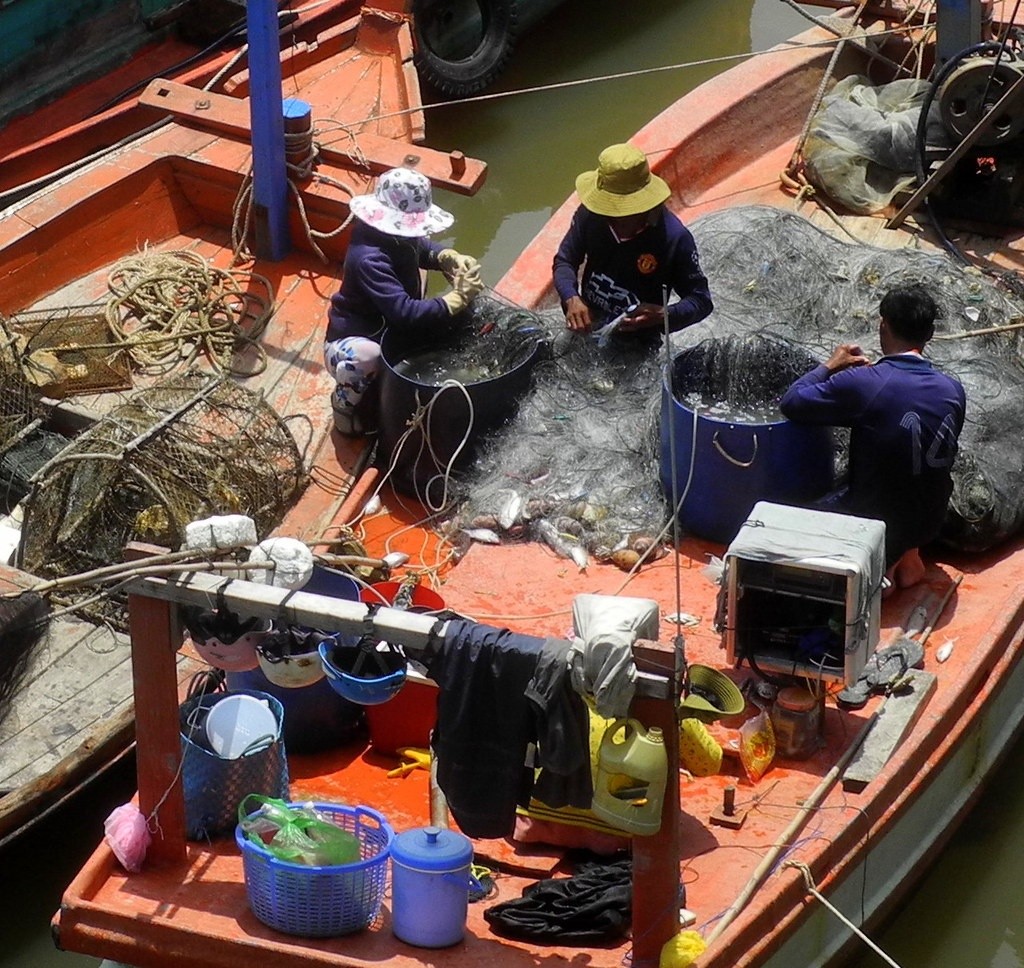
[443,264,482,315]
[438,248,485,291]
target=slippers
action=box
[835,638,924,709]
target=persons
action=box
[779,285,966,597]
[323,166,484,436]
[552,143,715,367]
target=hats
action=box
[349,167,455,236]
[677,663,745,726]
[575,143,672,217]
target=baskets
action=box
[234,801,394,938]
[178,689,293,841]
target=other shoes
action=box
[331,391,365,440]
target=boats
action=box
[47,0,1023,967]
[0,0,492,895]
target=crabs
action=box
[664,612,703,627]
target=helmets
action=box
[189,608,272,672]
[254,624,331,688]
[318,631,408,706]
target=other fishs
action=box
[459,487,591,576]
[936,633,961,663]
[900,606,927,639]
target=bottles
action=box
[590,717,669,837]
[770,687,821,761]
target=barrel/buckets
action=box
[186,694,277,757]
[224,565,447,753]
[390,826,483,948]
[376,322,540,476]
[658,343,834,542]
